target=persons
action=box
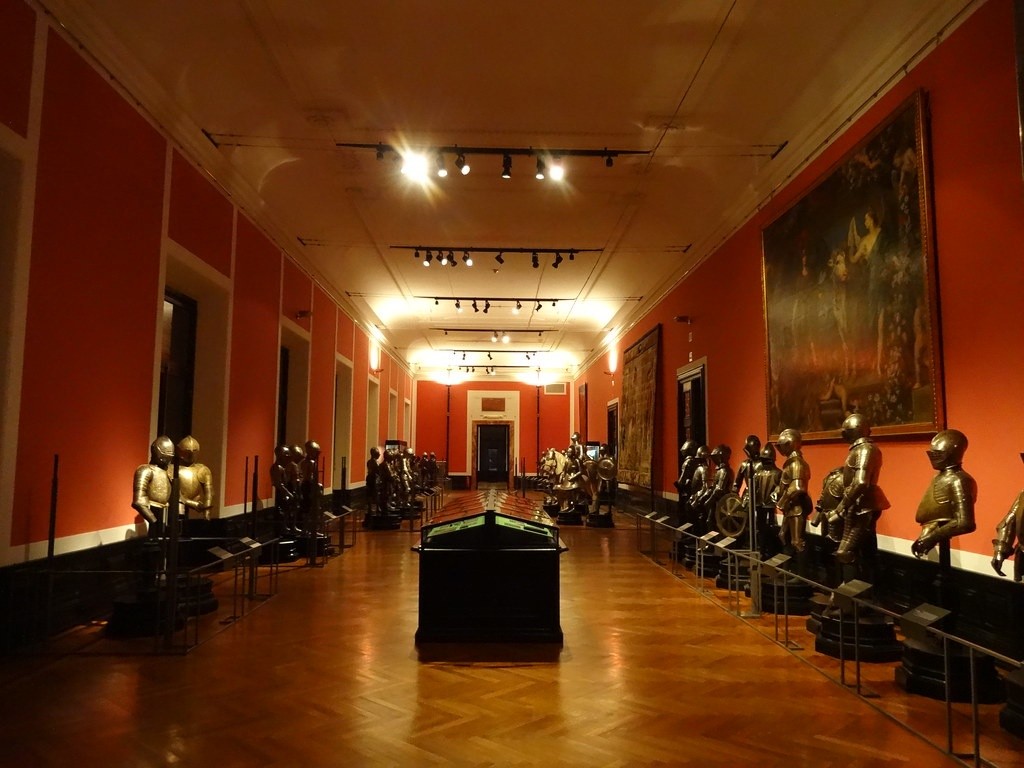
[541,433,612,514]
[366,447,439,510]
[270,441,327,536]
[992,453,1024,584]
[673,429,813,584]
[912,429,978,560]
[814,413,891,588]
[132,436,214,588]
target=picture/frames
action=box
[545,383,567,395]
[754,86,948,446]
[579,383,587,444]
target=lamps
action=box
[428,328,560,375]
[415,296,578,313]
[389,246,605,268]
[336,142,654,180]
